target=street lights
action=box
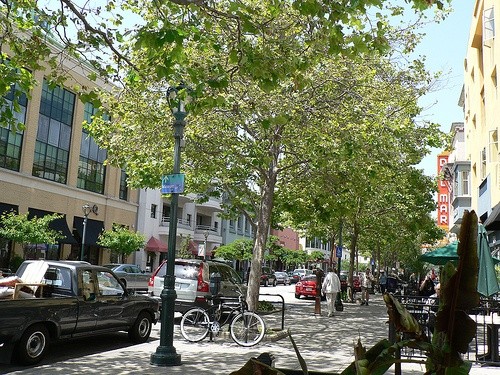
[80,203,99,260]
[203,231,210,260]
[152,78,182,368]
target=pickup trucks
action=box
[0,260,160,369]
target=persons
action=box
[358,270,387,305]
[322,267,341,318]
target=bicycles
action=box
[180,289,267,348]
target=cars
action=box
[275,272,289,285]
[99,263,152,291]
[294,269,318,281]
[295,275,326,301]
[246,266,277,287]
[341,269,388,293]
[288,272,300,283]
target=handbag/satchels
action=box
[336,293,343,312]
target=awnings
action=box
[188,239,212,256]
[146,236,168,252]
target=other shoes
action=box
[328,312,334,317]
[361,302,368,306]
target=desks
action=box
[470,315,500,368]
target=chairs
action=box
[0,260,50,299]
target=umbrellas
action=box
[420,220,500,317]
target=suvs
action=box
[148,259,250,318]
